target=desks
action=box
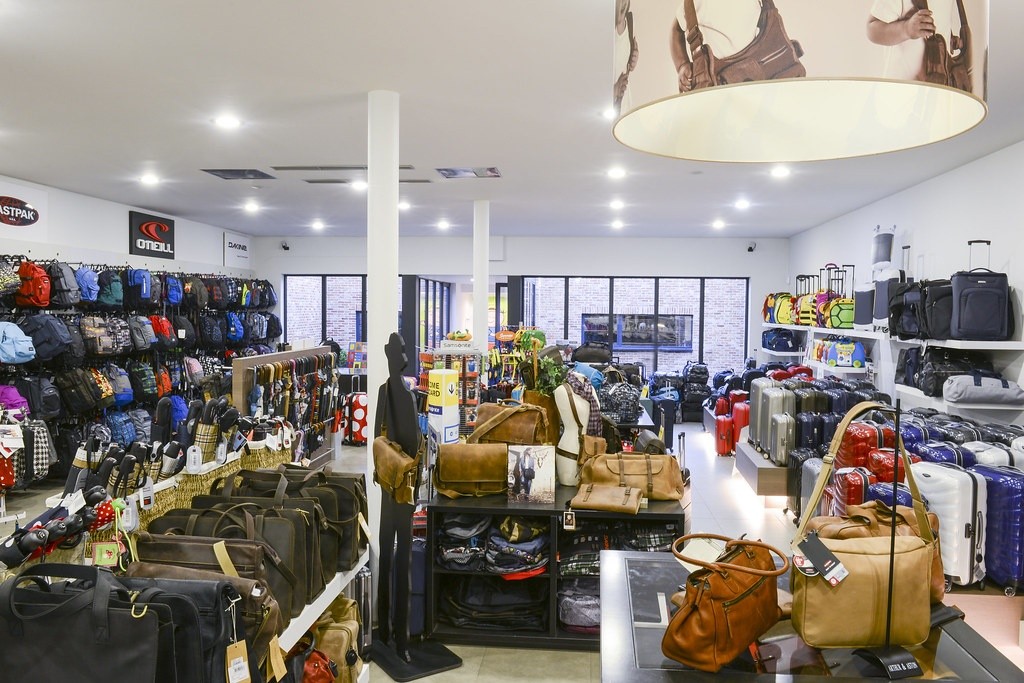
[600,549,1024,682]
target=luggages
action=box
[759,239,1015,341]
[702,345,1024,598]
[678,431,692,548]
[341,374,368,447]
[651,378,675,454]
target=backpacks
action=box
[0,254,282,494]
[318,337,340,364]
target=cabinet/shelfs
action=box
[759,320,1024,413]
[424,495,686,653]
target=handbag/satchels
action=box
[790,400,946,647]
[571,341,711,423]
[0,463,372,683]
[524,468,535,479]
[665,0,807,92]
[661,532,789,674]
[761,328,799,352]
[894,346,1024,405]
[813,334,867,368]
[910,0,973,93]
[373,379,426,505]
[431,346,684,515]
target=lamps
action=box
[612,1,989,163]
